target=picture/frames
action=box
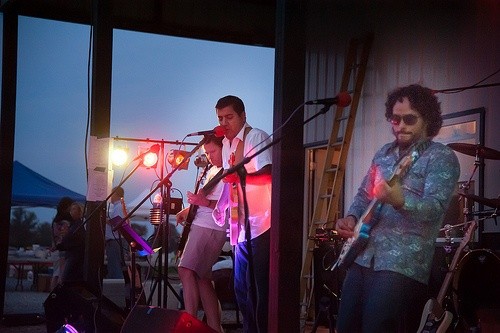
[426,107,485,246]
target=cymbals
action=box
[447,142,500,160]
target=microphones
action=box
[305,92,351,108]
[133,144,160,161]
[187,126,226,137]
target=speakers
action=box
[43,279,219,333]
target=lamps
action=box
[112,138,190,170]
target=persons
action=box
[105,187,127,279]
[49,197,85,294]
[336,85,460,333]
[213,95,273,333]
[177,130,230,333]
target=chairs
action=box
[453,249,500,322]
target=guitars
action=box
[331,152,414,272]
[174,164,208,263]
[416,220,477,333]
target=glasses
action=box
[389,114,422,126]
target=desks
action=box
[6,258,54,292]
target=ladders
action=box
[300,29,377,323]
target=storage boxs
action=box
[37,273,54,292]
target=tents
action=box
[11,159,87,206]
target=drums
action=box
[436,246,500,333]
[435,180,478,244]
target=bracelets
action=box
[208,200,211,207]
